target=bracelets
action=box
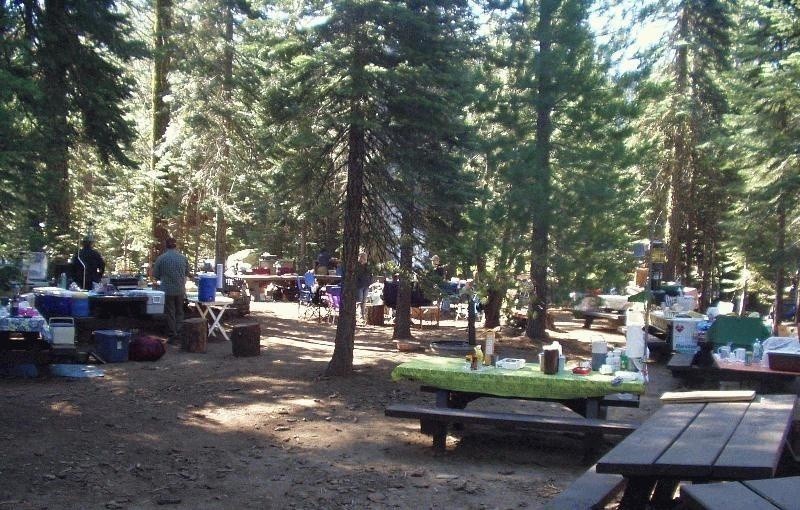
[660,284,679,294]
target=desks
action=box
[0,307,52,369]
[223,273,342,303]
[648,306,800,394]
[396,356,646,438]
[188,295,233,341]
[680,476,798,509]
[185,291,224,301]
[596,393,799,510]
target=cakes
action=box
[745,352,753,365]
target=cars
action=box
[718,346,745,363]
[591,341,606,372]
[491,354,498,366]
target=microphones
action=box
[364,305,384,326]
[183,317,209,353]
[232,324,260,355]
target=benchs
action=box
[385,403,641,456]
[572,310,627,329]
[618,326,702,381]
[545,465,625,508]
[420,384,640,420]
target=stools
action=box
[183,317,209,353]
[232,324,260,355]
[364,305,384,326]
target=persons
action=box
[152,238,189,343]
[380,255,485,315]
[355,252,369,321]
[71,234,106,290]
[315,249,330,286]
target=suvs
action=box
[194,273,218,302]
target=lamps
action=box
[380,280,442,330]
[48,316,77,364]
[296,275,342,324]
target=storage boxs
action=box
[95,330,130,362]
[50,323,75,344]
[34,287,165,318]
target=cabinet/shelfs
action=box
[750,338,761,362]
[605,350,628,370]
[473,345,483,369]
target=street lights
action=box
[128,336,165,362]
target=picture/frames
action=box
[223,273,342,303]
[0,307,52,369]
[188,295,233,341]
[185,291,224,301]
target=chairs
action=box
[48,316,77,364]
[296,275,342,324]
[380,280,442,330]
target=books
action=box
[34,287,165,318]
[50,323,75,344]
[95,330,130,362]
